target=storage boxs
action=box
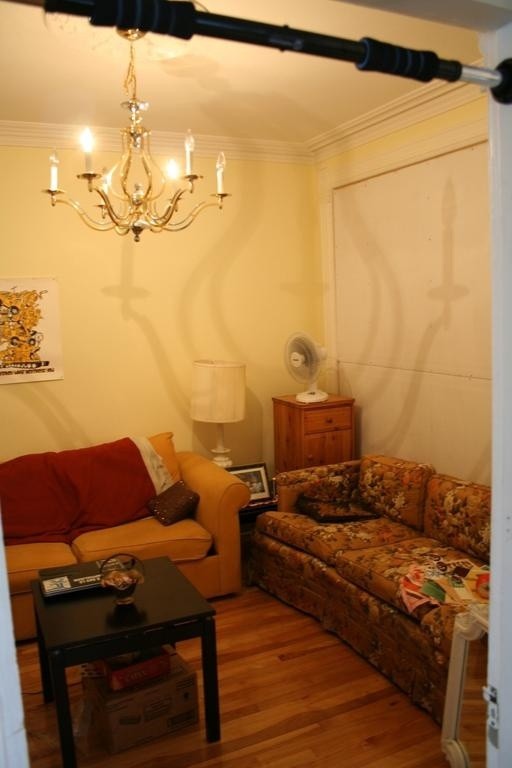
[80,643,200,754]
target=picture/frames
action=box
[226,462,272,505]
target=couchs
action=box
[1,433,249,642]
[247,454,493,728]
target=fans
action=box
[283,331,329,403]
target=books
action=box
[38,557,124,597]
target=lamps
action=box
[190,359,246,470]
[44,27,231,244]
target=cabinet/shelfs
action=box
[440,608,488,767]
[273,392,355,489]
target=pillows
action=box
[295,495,381,523]
[146,479,200,526]
